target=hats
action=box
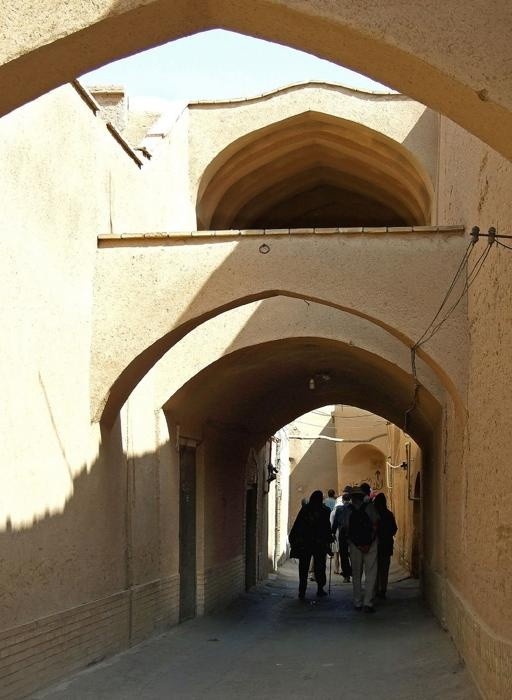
[348,486,366,496]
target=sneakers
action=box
[297,585,328,598]
[308,570,351,584]
[351,598,377,614]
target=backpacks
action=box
[346,500,376,555]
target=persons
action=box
[343,487,381,612]
[360,483,371,503]
[374,492,398,603]
[332,494,351,583]
[330,486,353,575]
[323,488,335,508]
[288,489,333,599]
